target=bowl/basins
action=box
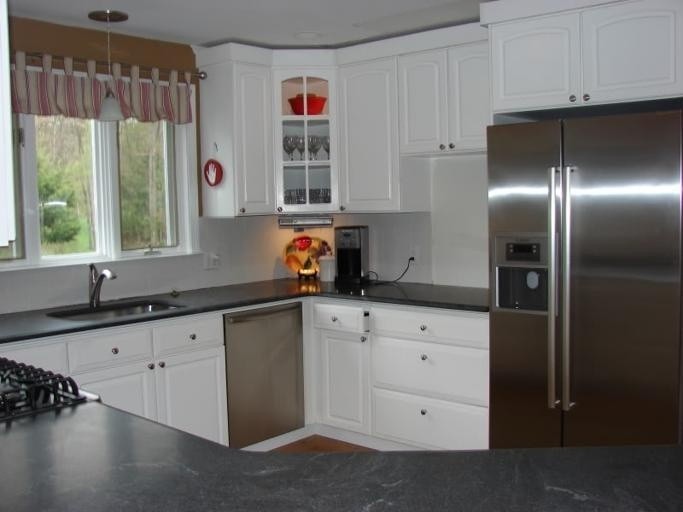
[287,97,326,114]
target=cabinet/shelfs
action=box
[310,299,370,436]
[236,60,273,216]
[489,3,682,112]
[371,306,490,454]
[275,65,337,215]
[66,316,229,453]
[338,58,398,212]
[397,40,490,152]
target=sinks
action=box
[45,300,183,321]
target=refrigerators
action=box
[487,109,682,447]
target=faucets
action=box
[88,263,117,308]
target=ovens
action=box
[223,303,305,449]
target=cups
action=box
[284,189,330,204]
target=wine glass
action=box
[283,135,329,161]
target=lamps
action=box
[88,10,128,121]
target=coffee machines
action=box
[333,225,369,287]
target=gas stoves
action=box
[0,357,100,423]
